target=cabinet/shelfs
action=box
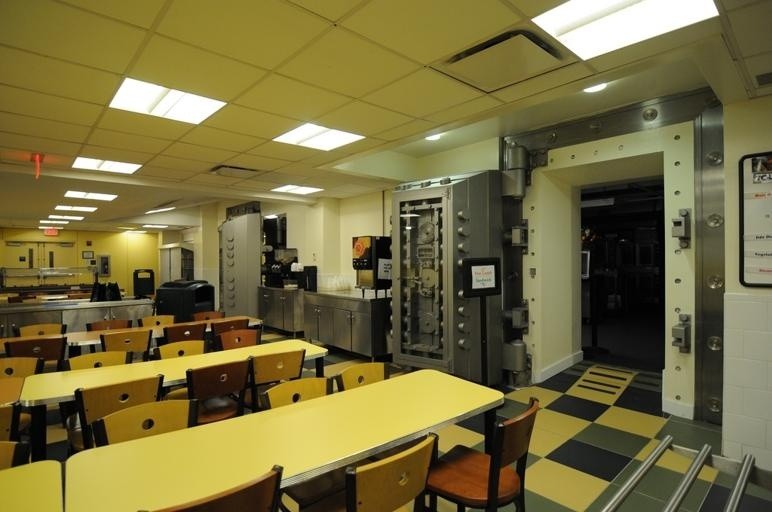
[62,304,154,332]
[333,308,372,357]
[257,287,295,332]
[304,304,333,346]
